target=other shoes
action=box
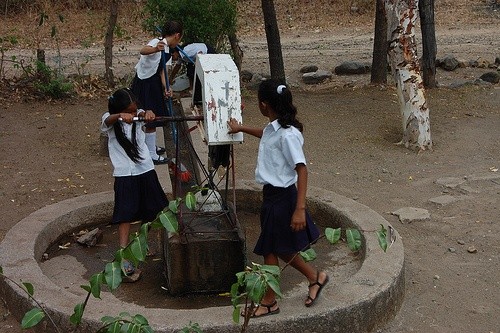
[156,145,166,154]
[152,155,168,164]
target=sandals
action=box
[121,261,134,275]
[306,270,329,306]
[241,302,280,318]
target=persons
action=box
[100,88,173,284]
[172,42,234,182]
[130,19,186,166]
[228,78,328,319]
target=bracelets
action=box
[296,208,305,210]
